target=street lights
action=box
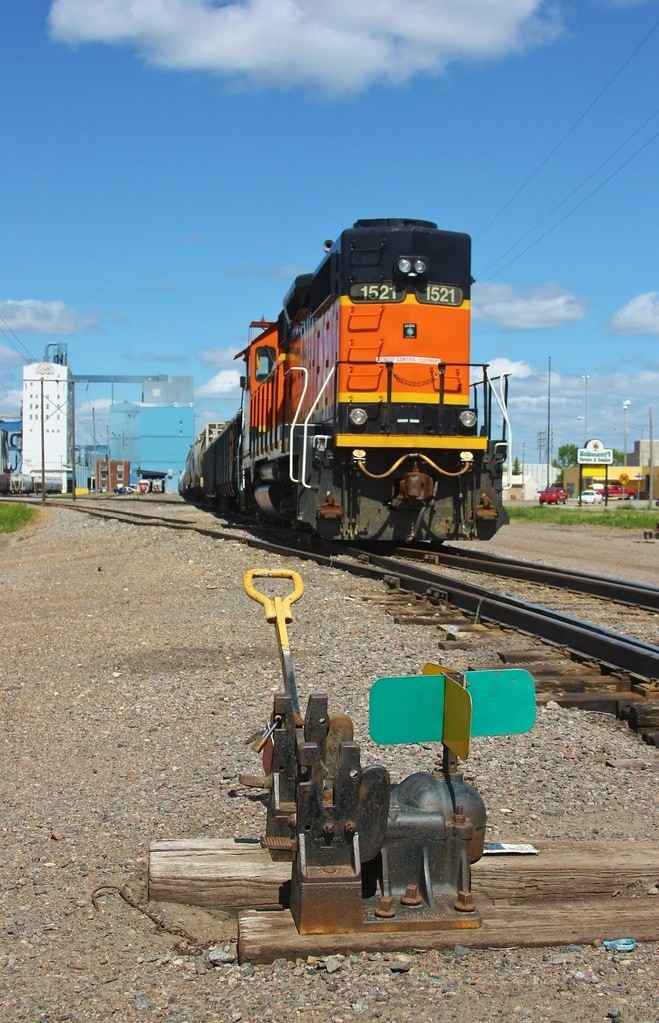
[578,416,587,443]
[582,368,590,444]
[623,400,631,473]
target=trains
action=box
[178,217,511,546]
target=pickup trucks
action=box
[539,487,569,505]
[597,485,637,500]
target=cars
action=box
[114,487,136,495]
[578,490,603,504]
